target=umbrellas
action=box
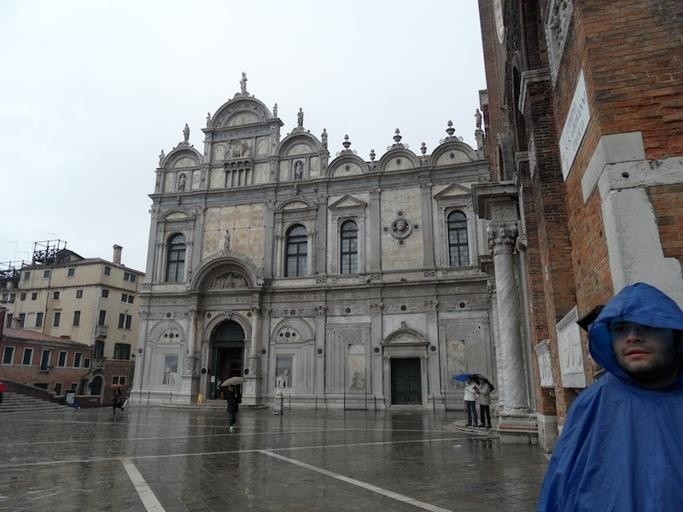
[109,382,125,390]
[219,375,248,395]
[469,373,494,393]
[454,372,475,382]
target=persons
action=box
[215,378,222,399]
[158,148,166,167]
[462,377,479,427]
[472,379,492,429]
[534,282,683,512]
[112,389,125,415]
[296,106,303,127]
[474,107,483,129]
[179,174,186,192]
[182,123,189,141]
[321,128,328,145]
[225,384,242,431]
[296,161,303,179]
[239,71,247,95]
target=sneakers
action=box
[466,423,491,428]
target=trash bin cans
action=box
[197,393,204,405]
[64,390,75,407]
